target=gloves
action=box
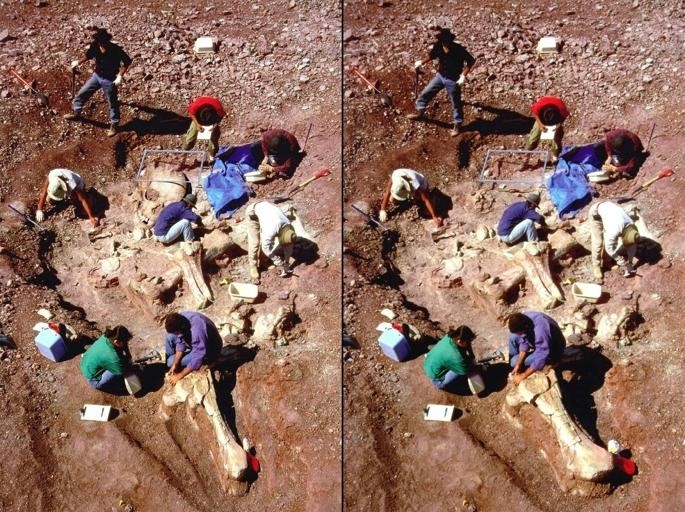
[113,74,122,86]
[379,210,389,223]
[35,210,45,222]
[456,75,466,85]
[415,60,422,68]
[618,257,637,274]
[605,157,616,172]
[260,157,273,173]
[71,61,78,69]
[274,255,294,278]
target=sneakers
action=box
[407,113,423,119]
[594,265,603,278]
[451,125,461,137]
[107,123,117,137]
[63,112,81,120]
[175,160,186,171]
[249,265,260,279]
[518,161,528,171]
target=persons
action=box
[516,95,570,172]
[246,200,296,286]
[35,168,100,227]
[153,193,204,244]
[163,310,222,387]
[379,169,442,228]
[497,193,545,244]
[258,128,301,179]
[407,29,475,137]
[603,128,643,182]
[422,325,477,389]
[80,324,133,391]
[506,308,554,383]
[589,200,641,284]
[175,96,226,172]
[63,28,132,137]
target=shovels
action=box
[10,69,48,106]
[354,69,392,107]
[611,167,676,201]
[275,168,332,201]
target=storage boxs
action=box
[34,328,66,365]
[378,328,410,362]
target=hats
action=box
[196,104,217,126]
[180,193,198,209]
[48,178,68,202]
[390,174,413,202]
[612,136,632,155]
[436,29,456,43]
[93,29,112,42]
[622,224,640,248]
[519,193,541,209]
[538,104,561,125]
[279,225,298,248]
[268,134,287,153]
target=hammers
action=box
[279,269,287,278]
[477,350,505,363]
[133,349,162,364]
